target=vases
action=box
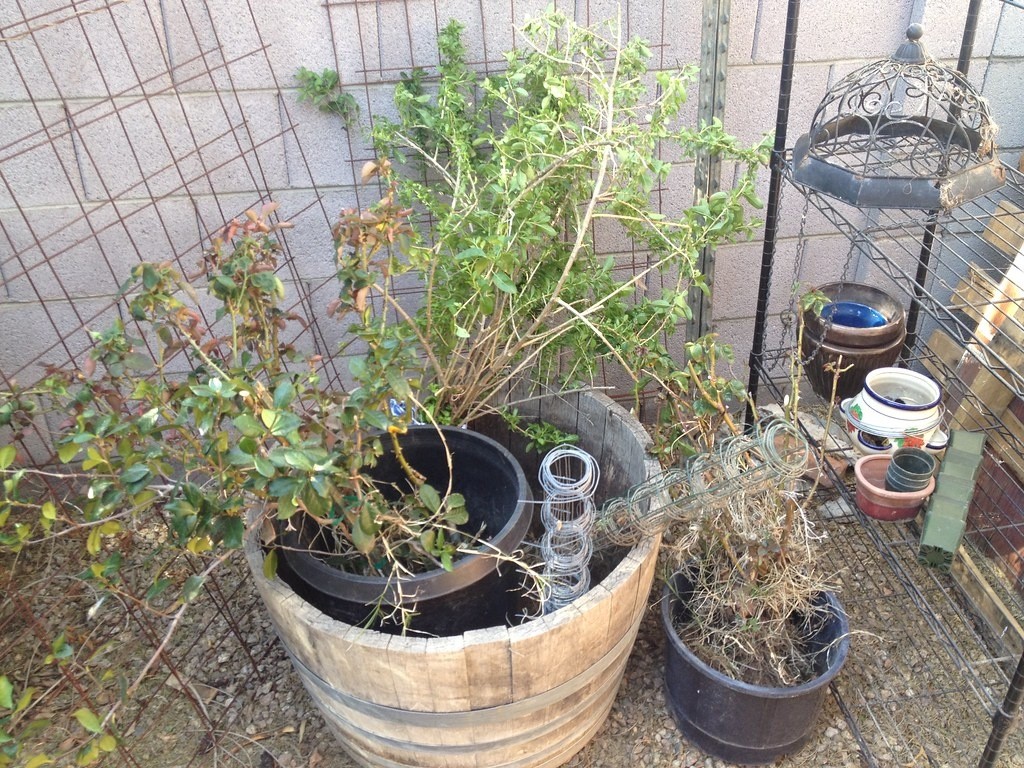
[798,275,989,576]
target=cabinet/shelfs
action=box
[738,13,1024,768]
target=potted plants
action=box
[0,0,785,768]
[656,297,853,767]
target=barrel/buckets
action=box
[854,446,937,523]
[233,361,668,768]
